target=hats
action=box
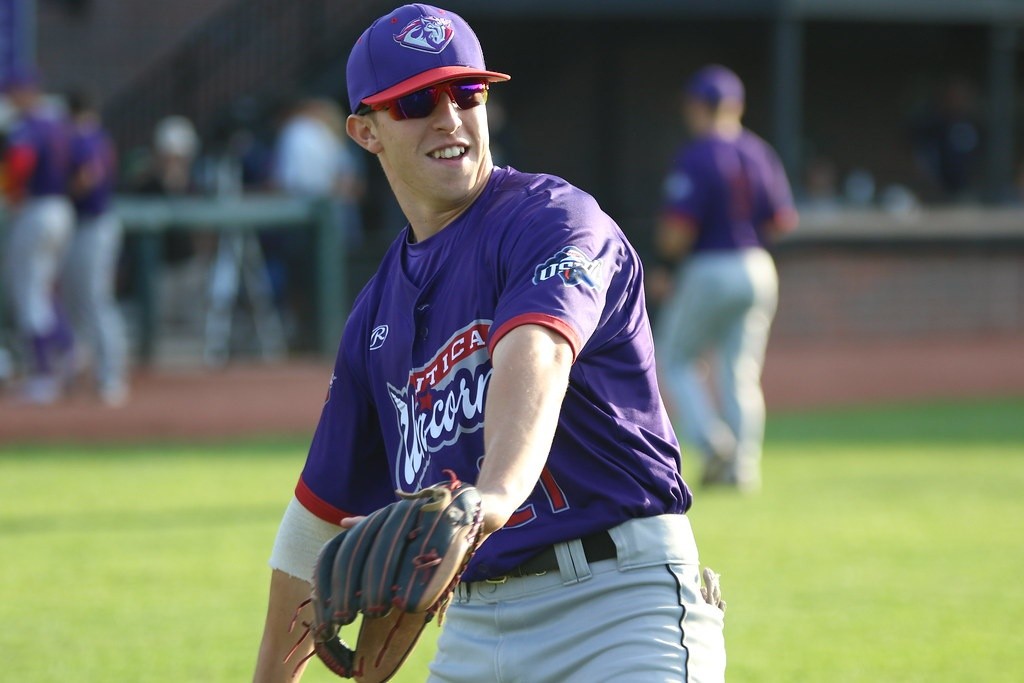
[680,63,749,104]
[346,3,511,130]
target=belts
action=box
[483,530,620,587]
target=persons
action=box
[252,5,726,683]
[0,65,989,494]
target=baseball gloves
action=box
[308,477,484,683]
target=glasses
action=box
[357,85,490,121]
